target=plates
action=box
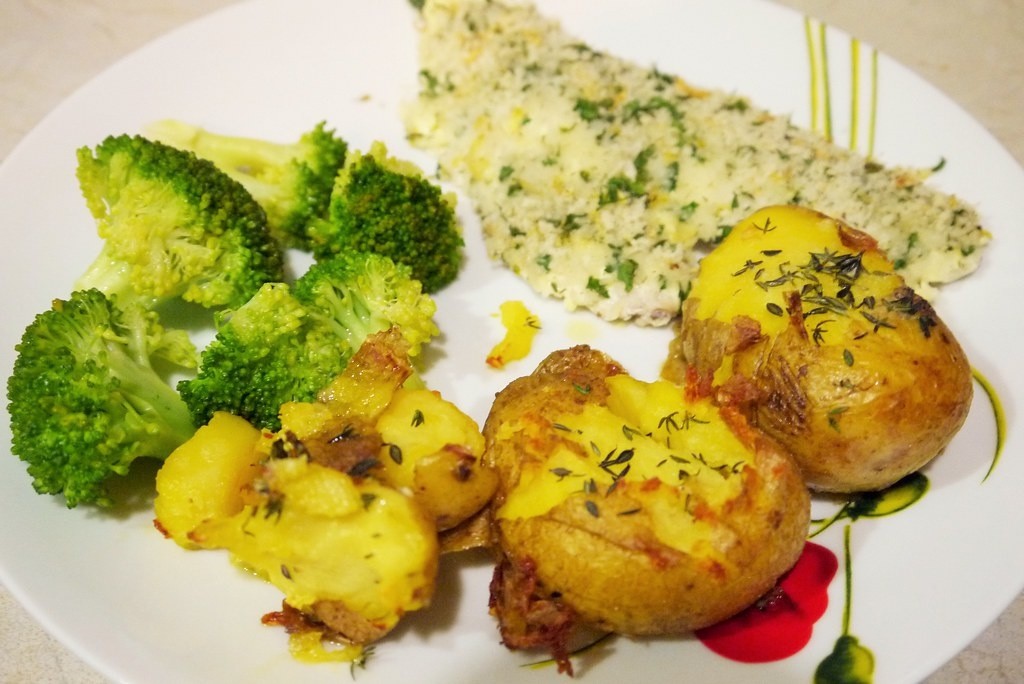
[0,0,1024,684]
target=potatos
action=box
[151,207,976,637]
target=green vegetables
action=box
[416,44,946,521]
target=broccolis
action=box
[5,118,466,510]
